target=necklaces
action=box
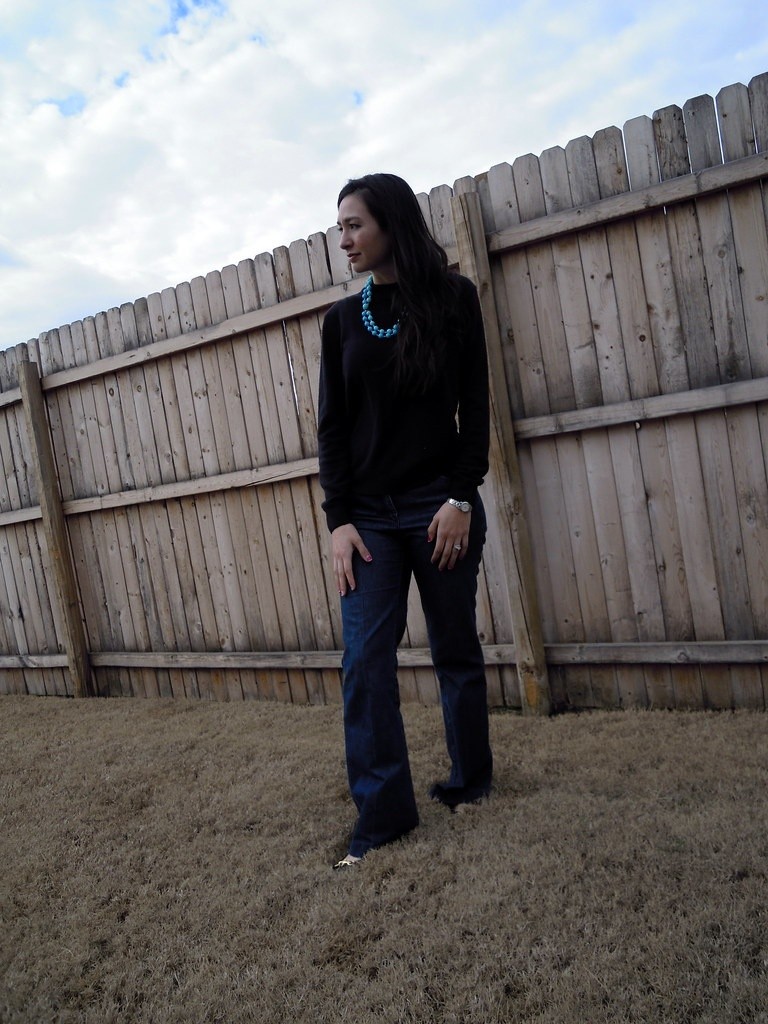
[362,272,401,341]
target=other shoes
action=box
[332,853,362,869]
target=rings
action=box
[455,545,462,552]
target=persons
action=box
[318,174,492,867]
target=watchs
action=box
[447,498,474,514]
